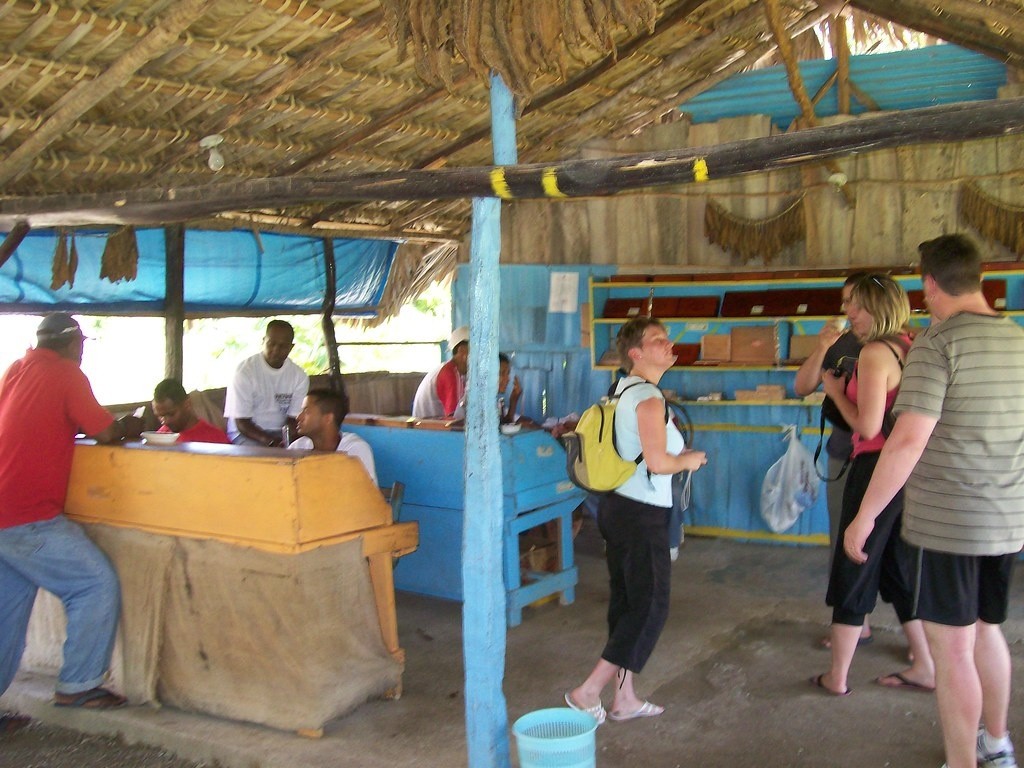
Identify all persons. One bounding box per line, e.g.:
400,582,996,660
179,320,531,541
844,233,1023,768
284,387,378,490
452,352,523,426
151,379,234,444
568,318,709,719
809,273,937,695
223,319,311,449
0,315,147,708
793,270,877,645
412,340,470,418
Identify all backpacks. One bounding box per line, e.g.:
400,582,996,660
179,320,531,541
562,381,670,491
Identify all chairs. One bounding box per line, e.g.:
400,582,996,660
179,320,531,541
378,481,409,570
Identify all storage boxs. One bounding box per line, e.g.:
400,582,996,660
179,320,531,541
699,324,834,400
515,538,579,607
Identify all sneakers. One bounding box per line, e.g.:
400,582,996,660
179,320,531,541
975,723,1016,768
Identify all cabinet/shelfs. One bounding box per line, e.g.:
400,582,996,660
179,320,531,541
586,262,1024,434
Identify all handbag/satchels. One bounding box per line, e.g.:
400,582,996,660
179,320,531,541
821,357,858,434
762,430,824,533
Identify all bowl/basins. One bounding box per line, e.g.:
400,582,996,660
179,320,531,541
140,431,181,444
501,423,521,435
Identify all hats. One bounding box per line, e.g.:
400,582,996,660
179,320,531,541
37,313,88,343
449,326,470,350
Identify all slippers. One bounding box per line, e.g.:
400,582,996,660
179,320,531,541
608,699,663,722
0,712,32,735
809,675,852,698
565,692,606,726
874,673,936,692
54,688,129,711
818,632,872,649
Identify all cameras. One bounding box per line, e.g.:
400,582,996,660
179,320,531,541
835,357,858,382
